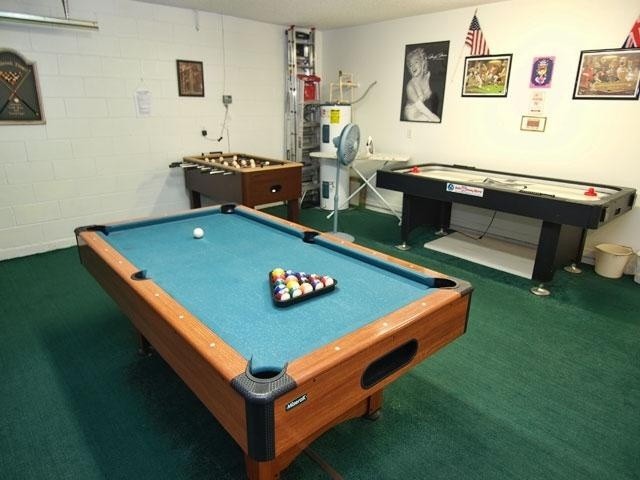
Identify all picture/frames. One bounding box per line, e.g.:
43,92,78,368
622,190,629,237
0,46,48,125
461,53,514,98
572,47,640,100
176,59,205,97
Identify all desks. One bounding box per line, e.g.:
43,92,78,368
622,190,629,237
374,160,637,297
73,203,476,480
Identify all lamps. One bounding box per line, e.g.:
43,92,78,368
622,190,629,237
0,0,97,29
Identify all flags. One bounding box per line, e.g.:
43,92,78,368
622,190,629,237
464,14,490,56
622,15,640,48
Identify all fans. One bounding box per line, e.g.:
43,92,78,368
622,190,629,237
323,123,360,243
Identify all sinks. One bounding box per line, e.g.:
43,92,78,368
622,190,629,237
349,156,408,179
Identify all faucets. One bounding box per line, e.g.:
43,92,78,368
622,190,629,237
366,135,374,156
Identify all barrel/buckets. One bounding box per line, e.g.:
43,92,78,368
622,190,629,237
593,243,633,279
633,250,640,284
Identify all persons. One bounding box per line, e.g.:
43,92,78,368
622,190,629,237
403,48,441,122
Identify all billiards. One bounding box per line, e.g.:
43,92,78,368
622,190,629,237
271,268,334,301
193,228,204,238
